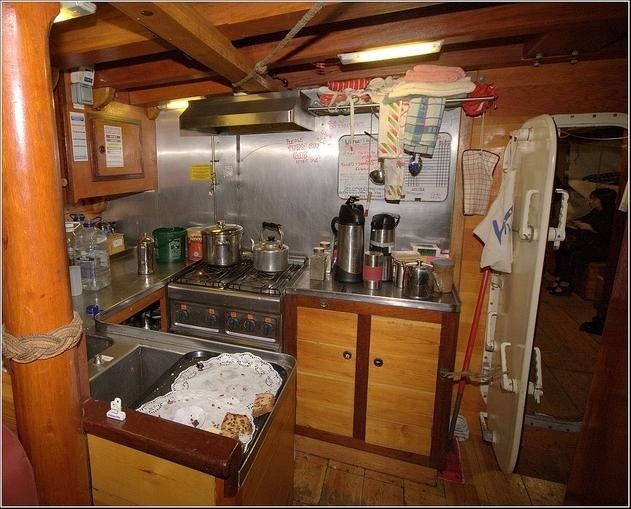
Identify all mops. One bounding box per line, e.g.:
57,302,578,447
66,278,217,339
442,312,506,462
437,267,491,485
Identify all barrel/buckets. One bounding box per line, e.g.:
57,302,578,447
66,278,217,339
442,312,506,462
152,226,187,263
68,212,113,293
186,227,206,263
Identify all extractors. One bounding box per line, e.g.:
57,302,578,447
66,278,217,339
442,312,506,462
178,89,317,137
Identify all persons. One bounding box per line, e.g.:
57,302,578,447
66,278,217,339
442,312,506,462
546,188,616,294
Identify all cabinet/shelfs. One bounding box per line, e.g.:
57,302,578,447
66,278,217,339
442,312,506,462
286,293,457,472
90,366,293,506
54,73,157,205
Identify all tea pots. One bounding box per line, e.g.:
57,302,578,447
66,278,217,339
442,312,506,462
331,195,366,284
250,221,289,273
368,213,402,282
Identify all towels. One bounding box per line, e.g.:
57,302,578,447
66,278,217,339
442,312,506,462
308,64,476,201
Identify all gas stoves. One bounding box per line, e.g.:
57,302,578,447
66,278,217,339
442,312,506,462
167,252,309,353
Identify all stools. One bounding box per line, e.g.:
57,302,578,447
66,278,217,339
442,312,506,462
578,262,612,303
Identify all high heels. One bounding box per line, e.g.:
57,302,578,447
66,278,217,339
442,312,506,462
546,280,572,296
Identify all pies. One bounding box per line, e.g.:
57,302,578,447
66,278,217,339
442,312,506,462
220,412,252,444
252,394,275,418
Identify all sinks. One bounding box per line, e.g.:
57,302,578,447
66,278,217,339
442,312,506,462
83,332,115,363
88,344,185,410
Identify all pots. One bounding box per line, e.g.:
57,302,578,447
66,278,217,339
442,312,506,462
200,219,245,268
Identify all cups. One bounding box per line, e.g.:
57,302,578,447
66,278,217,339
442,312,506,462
68,264,83,298
363,251,384,291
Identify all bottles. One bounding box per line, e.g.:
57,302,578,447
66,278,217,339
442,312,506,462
137,230,157,276
309,240,332,281
431,259,456,294
65,213,113,291
100,221,126,256
